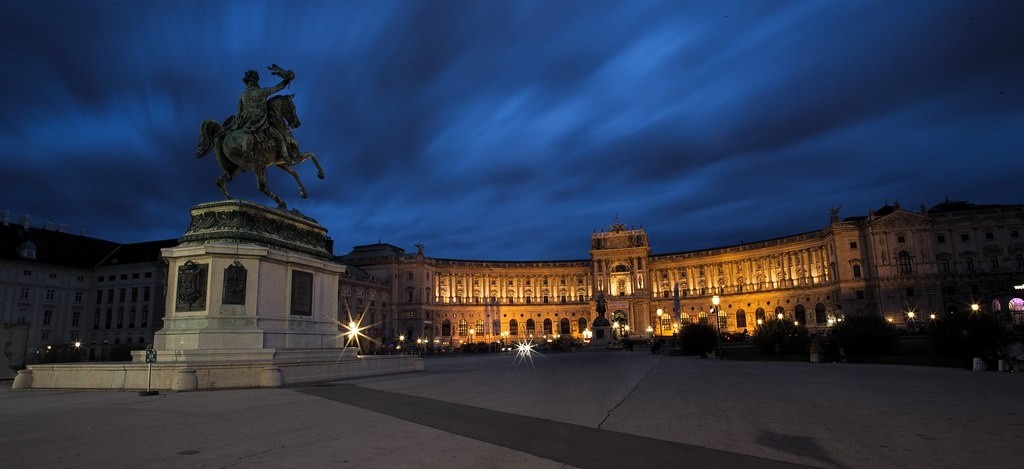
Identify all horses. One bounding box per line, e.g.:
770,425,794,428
196,93,324,211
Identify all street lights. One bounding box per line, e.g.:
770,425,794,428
656,308,665,351
500,330,511,345
711,294,722,339
907,312,917,333
469,328,475,344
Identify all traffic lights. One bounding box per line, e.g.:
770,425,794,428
620,323,625,337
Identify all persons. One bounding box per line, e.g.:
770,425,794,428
231,69,295,164
596,294,607,316
